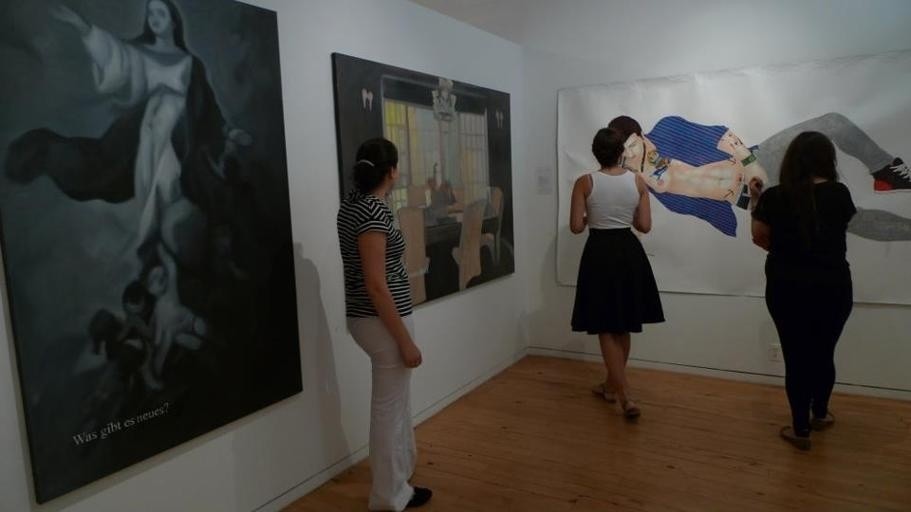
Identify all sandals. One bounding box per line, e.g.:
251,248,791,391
592,384,618,404
622,399,641,418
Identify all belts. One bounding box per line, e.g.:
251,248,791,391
737,185,750,210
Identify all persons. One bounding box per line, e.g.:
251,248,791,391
337,137,433,512
609,116,911,242
751,130,858,451
569,127,666,420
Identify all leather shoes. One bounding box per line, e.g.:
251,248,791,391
811,411,835,432
408,487,433,508
780,426,811,451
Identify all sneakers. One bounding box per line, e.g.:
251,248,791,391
872,158,911,192
